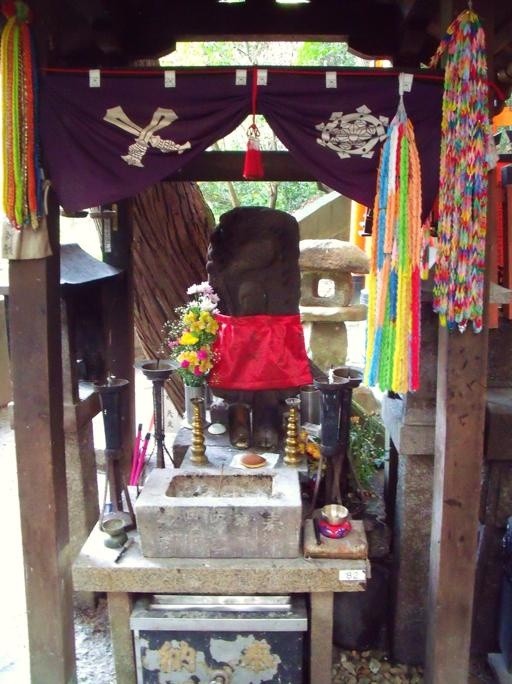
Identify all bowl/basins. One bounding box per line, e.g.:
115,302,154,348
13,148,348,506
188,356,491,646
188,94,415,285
321,502,350,526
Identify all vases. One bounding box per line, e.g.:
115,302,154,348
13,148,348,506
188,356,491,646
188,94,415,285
183,383,209,430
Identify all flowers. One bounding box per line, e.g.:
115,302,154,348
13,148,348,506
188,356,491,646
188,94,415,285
160,281,221,385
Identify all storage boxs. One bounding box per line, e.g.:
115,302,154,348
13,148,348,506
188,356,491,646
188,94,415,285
129,595,308,684
